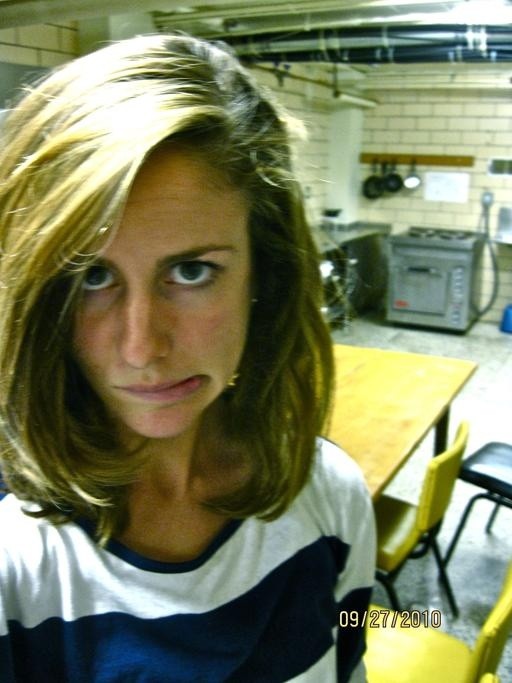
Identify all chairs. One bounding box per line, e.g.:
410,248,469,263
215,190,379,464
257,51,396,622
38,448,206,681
363,420,512,682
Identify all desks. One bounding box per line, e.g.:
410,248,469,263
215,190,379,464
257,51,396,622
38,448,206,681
315,345,480,503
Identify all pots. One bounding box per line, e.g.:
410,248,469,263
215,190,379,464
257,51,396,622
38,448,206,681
360,158,427,200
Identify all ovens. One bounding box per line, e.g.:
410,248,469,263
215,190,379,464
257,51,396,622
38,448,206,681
390,263,451,318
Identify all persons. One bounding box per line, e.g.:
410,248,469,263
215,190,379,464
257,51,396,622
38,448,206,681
0,32,383,681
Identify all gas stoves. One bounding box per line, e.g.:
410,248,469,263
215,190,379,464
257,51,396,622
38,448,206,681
404,226,473,240
